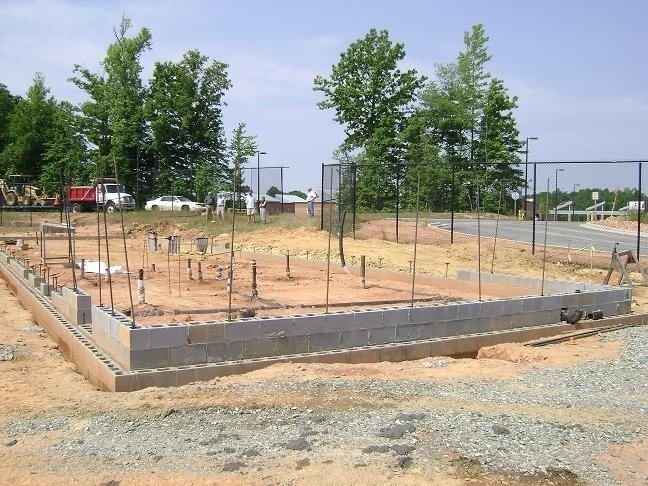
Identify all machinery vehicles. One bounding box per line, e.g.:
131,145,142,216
0,174,57,207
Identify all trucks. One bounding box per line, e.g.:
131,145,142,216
68,176,137,214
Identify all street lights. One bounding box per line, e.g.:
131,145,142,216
522,135,540,218
553,166,565,220
257,151,267,212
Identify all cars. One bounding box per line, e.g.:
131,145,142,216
144,195,206,213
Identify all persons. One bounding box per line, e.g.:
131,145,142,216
204,192,214,222
305,188,319,218
258,197,269,223
217,193,226,222
245,190,257,223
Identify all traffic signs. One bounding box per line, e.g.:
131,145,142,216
572,181,581,220
